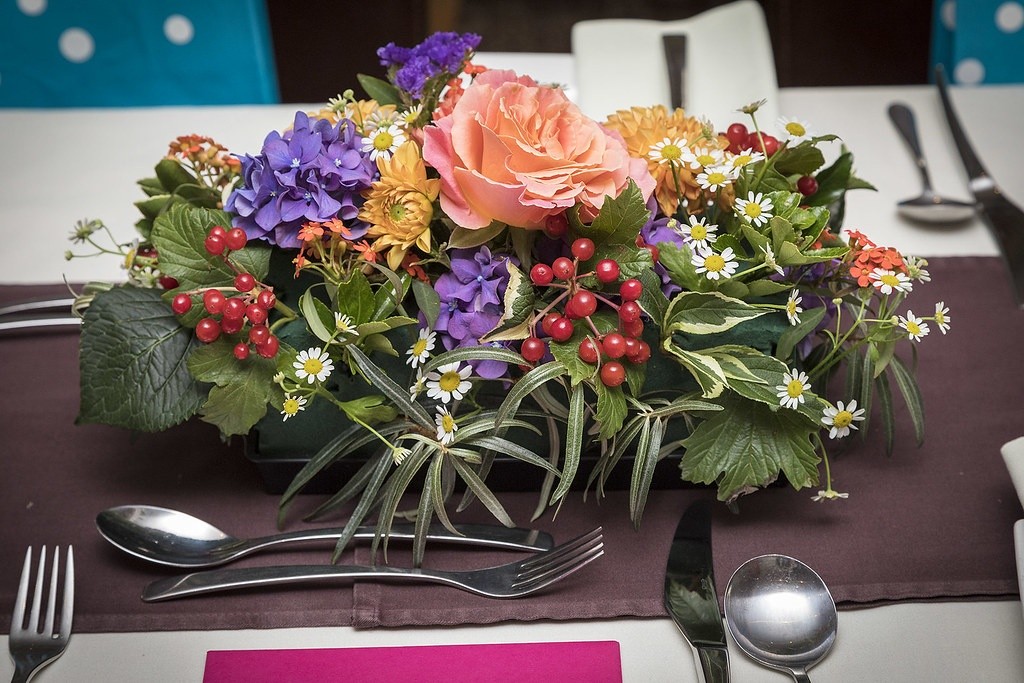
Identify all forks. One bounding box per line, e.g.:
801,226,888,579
9,544,75,683
142,525,605,604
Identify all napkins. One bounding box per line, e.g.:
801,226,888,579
469,1,781,138
0,255,1024,637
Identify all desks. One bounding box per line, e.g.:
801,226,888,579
0,85,1024,683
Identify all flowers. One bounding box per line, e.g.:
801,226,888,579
63,30,951,570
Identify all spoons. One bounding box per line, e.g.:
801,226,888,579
887,102,983,222
723,553,838,683
92,505,555,569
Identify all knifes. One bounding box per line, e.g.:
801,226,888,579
937,71,1024,306
663,501,732,683
